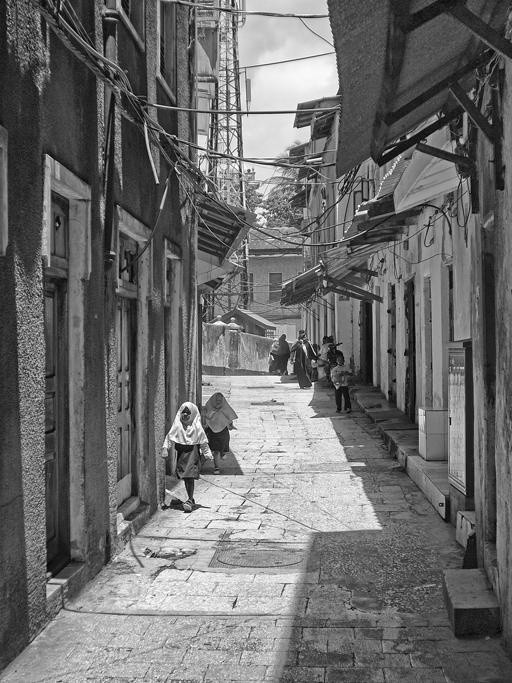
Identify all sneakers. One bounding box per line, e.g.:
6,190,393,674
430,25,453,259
213,454,227,474
335,406,351,413
183,498,196,511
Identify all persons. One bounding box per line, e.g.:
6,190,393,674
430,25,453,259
160,400,214,512
196,392,239,476
330,350,354,414
269,333,290,377
309,335,343,385
288,328,318,389
